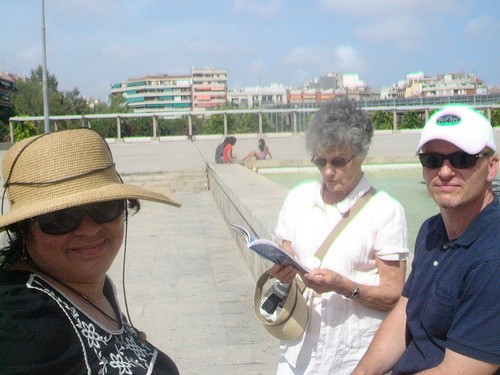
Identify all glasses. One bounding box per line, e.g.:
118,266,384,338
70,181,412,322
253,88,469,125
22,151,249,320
38,198,124,235
417,150,493,168
310,150,356,168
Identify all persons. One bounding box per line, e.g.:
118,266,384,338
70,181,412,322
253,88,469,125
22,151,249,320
0,130,185,375
223,137,239,165
350,103,500,375
243,139,273,161
269,99,410,375
215,137,230,163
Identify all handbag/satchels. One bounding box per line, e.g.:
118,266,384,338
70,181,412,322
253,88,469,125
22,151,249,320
254,267,311,342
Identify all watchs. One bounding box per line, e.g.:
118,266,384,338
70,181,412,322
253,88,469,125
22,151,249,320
349,282,359,299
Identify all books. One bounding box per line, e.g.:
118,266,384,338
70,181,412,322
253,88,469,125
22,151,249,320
229,222,311,275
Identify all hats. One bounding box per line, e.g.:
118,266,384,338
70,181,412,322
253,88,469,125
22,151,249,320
0,128,182,231
414,105,497,155
